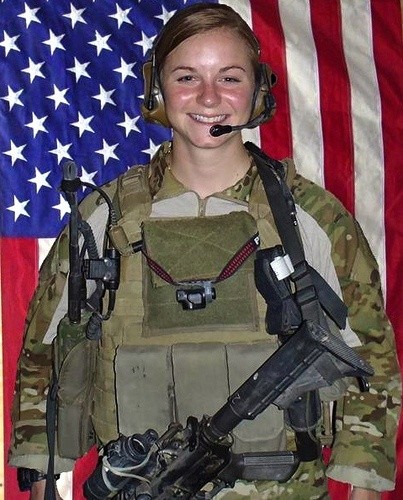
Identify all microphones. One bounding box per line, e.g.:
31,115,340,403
209,108,271,137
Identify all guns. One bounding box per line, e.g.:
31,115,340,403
83,318,375,500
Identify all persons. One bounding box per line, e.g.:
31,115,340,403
7,4,403,500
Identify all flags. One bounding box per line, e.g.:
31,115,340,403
0,0,403,500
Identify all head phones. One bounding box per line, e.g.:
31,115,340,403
138,3,278,130
